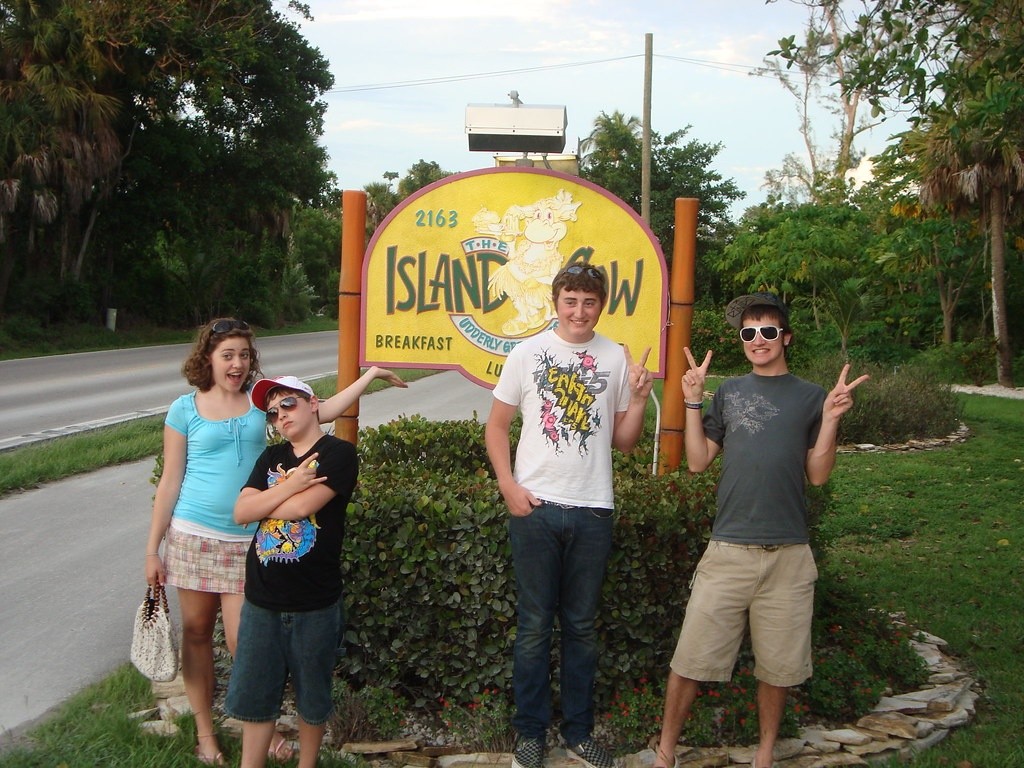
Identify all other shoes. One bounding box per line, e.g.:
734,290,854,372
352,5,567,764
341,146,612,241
566,737,618,768
512,736,543,768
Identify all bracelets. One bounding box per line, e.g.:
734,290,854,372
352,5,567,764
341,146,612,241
683,398,703,409
146,553,158,556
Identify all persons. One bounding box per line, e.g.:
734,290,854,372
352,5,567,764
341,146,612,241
223,375,359,768
653,291,869,768
485,261,653,768
145,318,409,766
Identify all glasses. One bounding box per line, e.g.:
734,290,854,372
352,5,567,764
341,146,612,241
562,265,604,282
739,325,784,341
265,396,301,423
209,320,250,337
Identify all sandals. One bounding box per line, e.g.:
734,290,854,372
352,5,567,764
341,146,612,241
267,736,294,765
197,732,225,768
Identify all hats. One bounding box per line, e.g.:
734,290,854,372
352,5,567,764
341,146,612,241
725,291,788,329
251,376,314,412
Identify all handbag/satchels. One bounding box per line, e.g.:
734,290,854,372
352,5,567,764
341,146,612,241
131,581,179,682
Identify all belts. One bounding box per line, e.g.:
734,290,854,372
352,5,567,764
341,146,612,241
721,541,794,553
543,501,580,510
